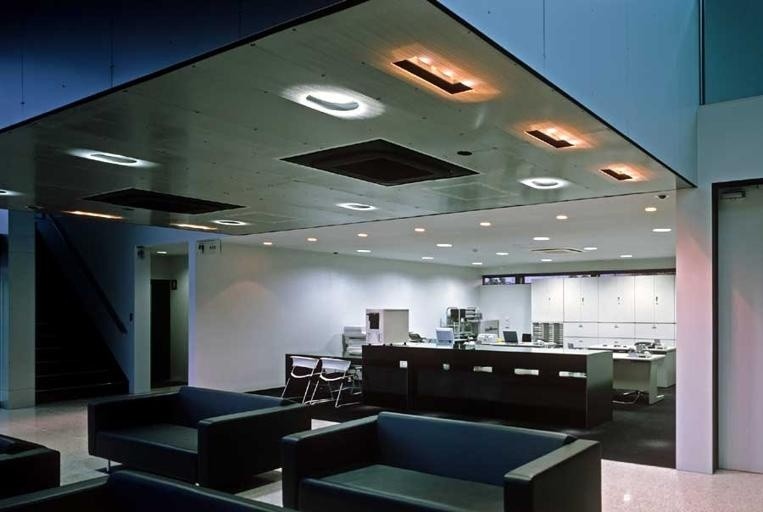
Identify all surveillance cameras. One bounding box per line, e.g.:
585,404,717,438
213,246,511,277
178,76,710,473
655,193,669,200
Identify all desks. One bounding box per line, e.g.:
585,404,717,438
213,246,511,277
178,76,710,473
590,346,677,390
279,351,362,403
611,354,665,408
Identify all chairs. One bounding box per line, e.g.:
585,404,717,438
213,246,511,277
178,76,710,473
279,354,321,405
309,356,360,407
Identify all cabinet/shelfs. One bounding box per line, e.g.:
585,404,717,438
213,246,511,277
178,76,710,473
445,306,479,343
359,337,614,429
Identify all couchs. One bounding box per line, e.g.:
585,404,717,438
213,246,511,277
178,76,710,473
2,467,291,512
279,405,601,512
0,428,62,497
81,383,311,491
